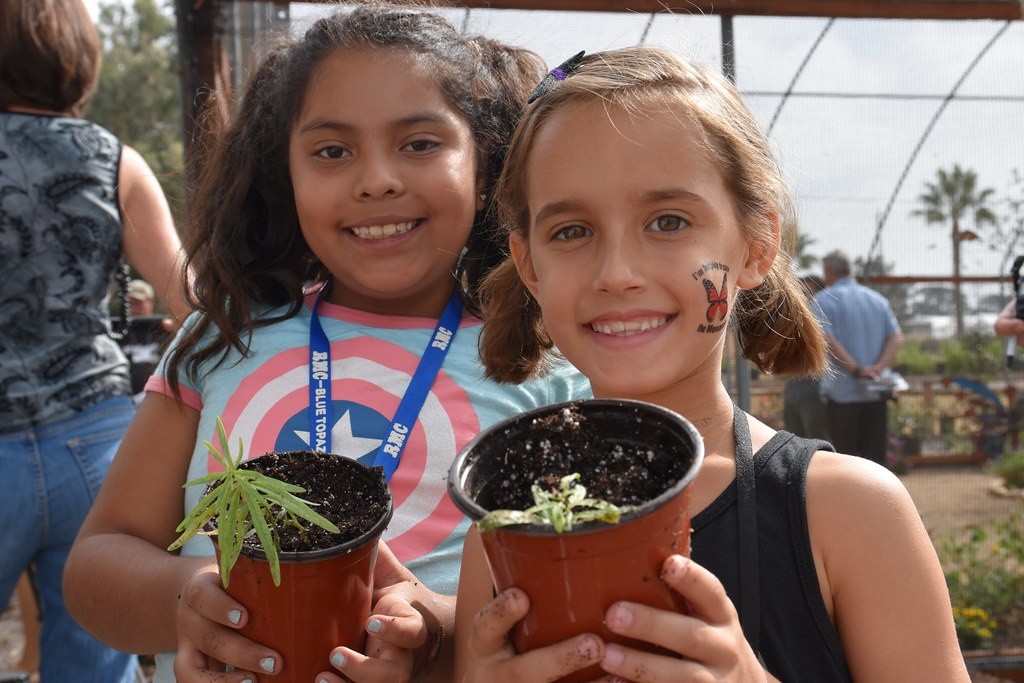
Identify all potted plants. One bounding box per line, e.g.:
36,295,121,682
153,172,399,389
167,416,394,683
447,398,708,655
941,378,1024,459
896,408,935,455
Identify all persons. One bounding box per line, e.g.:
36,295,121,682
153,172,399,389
0,2,203,683
445,43,969,683
781,251,905,467
994,254,1024,351
63,9,595,681
